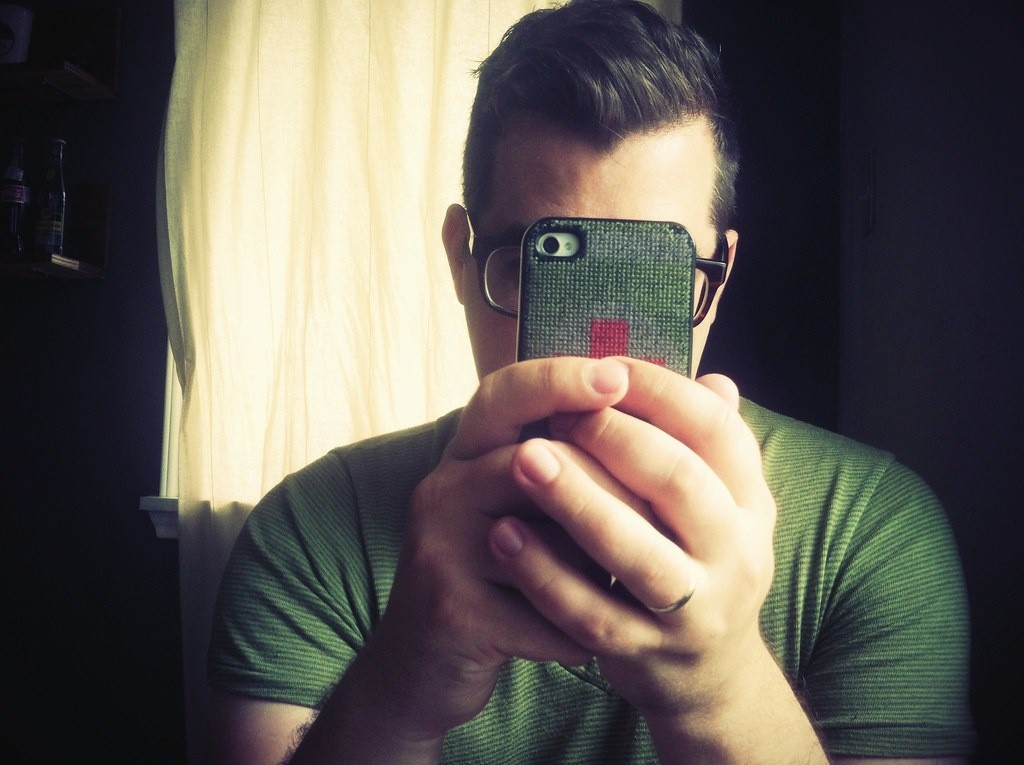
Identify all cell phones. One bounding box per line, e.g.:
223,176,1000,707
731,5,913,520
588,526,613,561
515,216,695,592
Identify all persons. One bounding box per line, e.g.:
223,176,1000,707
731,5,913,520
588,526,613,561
207,0,981,765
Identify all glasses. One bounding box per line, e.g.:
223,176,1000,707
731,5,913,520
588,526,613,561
464,208,730,329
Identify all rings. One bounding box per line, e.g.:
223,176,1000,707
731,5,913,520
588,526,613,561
648,554,699,612
